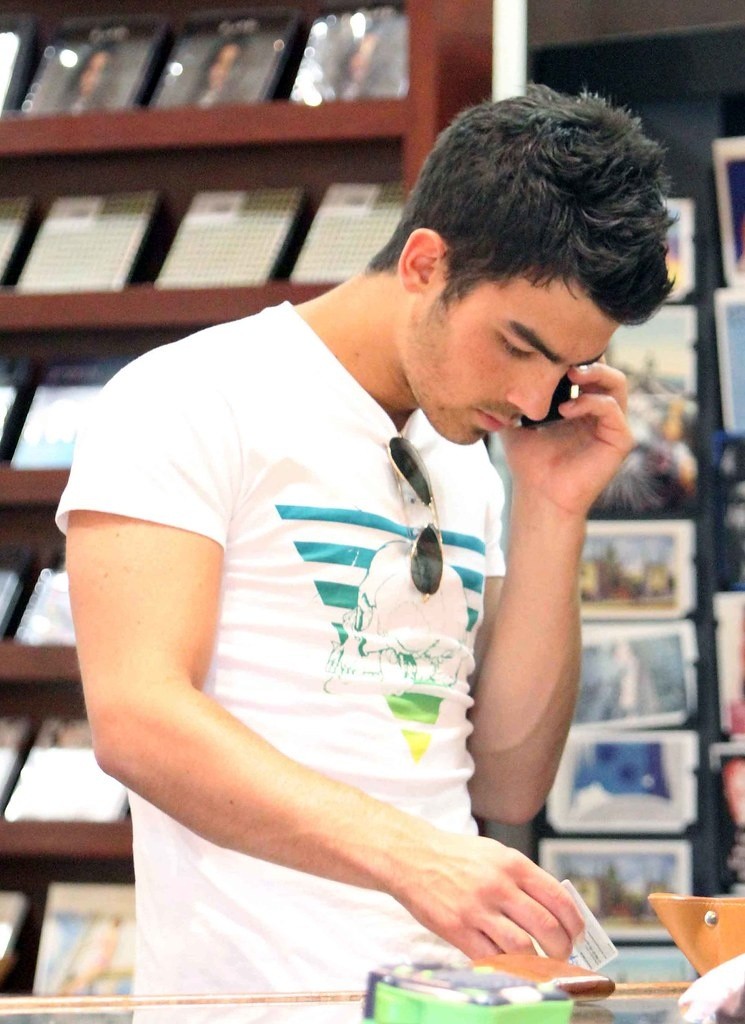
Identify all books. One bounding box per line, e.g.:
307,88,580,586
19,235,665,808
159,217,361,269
0,534,79,648
22,11,410,116
0,713,130,823
0,353,123,468
0,880,137,995
0,182,405,294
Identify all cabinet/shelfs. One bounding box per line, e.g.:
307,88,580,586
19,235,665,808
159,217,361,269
0,1,496,995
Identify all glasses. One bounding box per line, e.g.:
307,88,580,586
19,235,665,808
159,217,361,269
387,434,445,605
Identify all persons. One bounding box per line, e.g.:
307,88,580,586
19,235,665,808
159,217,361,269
67,45,111,108
331,21,392,97
193,36,251,106
56,93,672,997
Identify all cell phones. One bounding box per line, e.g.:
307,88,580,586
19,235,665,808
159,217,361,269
520,371,579,428
